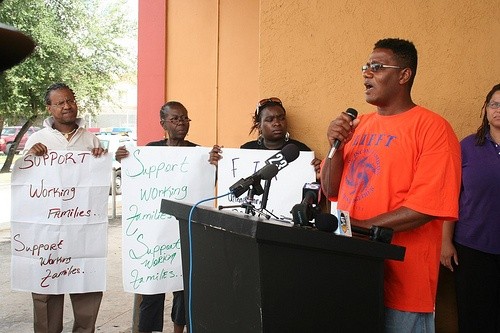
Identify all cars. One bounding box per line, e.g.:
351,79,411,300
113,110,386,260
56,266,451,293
86,126,134,137
0,126,35,154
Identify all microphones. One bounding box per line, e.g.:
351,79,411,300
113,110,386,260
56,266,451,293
315,210,394,244
229,144,300,191
233,164,278,198
292,182,322,224
328,108,358,159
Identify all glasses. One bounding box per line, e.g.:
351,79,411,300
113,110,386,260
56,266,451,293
161,118,191,125
362,63,408,74
257,97,283,115
485,101,500,109
49,99,76,107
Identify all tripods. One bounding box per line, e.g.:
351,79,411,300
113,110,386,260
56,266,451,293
218,189,270,220
253,179,280,220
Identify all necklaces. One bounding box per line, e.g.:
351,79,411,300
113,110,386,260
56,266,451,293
53,125,78,139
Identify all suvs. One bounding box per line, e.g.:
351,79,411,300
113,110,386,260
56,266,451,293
96,135,136,194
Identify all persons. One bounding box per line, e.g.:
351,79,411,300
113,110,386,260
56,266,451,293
320,38,462,333
439,83,500,333
115,103,217,333
22,83,107,333
209,98,324,208
